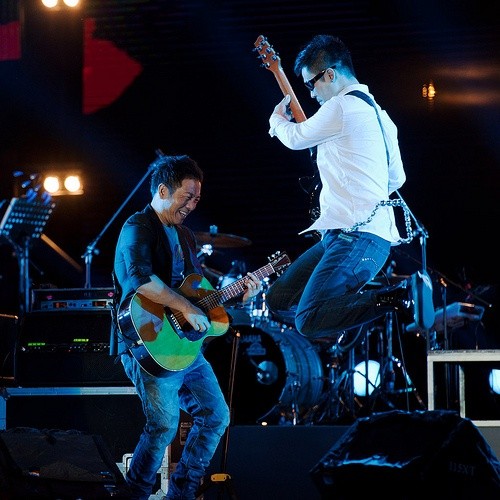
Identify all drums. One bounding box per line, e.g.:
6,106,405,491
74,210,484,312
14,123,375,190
202,322,324,425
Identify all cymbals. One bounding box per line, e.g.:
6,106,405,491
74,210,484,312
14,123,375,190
194,231,251,247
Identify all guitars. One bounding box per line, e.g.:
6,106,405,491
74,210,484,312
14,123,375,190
117,248,292,378
252,34,323,240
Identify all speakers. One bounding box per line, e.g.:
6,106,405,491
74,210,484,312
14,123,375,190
14,290,137,386
0,430,134,500
308,411,500,500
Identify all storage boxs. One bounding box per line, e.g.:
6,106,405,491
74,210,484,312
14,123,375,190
427,350,500,426
0,385,169,500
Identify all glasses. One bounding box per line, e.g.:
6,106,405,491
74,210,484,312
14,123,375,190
304,65,337,90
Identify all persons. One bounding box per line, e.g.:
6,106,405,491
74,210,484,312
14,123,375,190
263,34,437,337
110,152,264,500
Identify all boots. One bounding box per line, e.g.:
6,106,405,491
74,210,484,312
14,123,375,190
375,270,436,332
337,326,367,353
